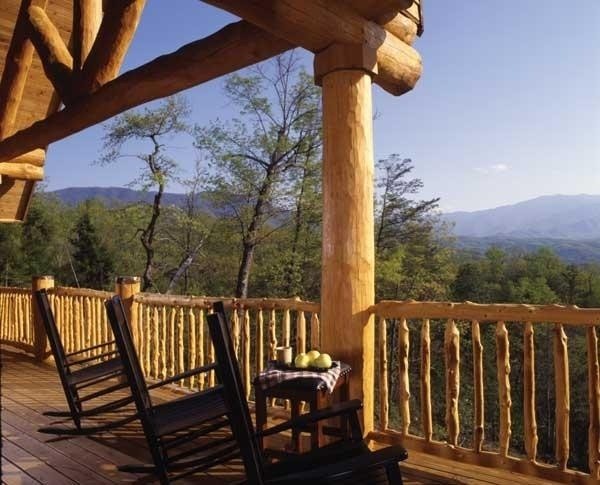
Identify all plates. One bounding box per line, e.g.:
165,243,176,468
268,359,339,373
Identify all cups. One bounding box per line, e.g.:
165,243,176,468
274,346,293,365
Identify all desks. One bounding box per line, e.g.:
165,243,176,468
253,359,350,457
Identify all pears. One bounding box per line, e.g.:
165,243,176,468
294,352,307,369
315,353,332,369
307,349,320,367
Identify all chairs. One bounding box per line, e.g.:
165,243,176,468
36,286,142,434
204,300,407,485
101,292,244,485
